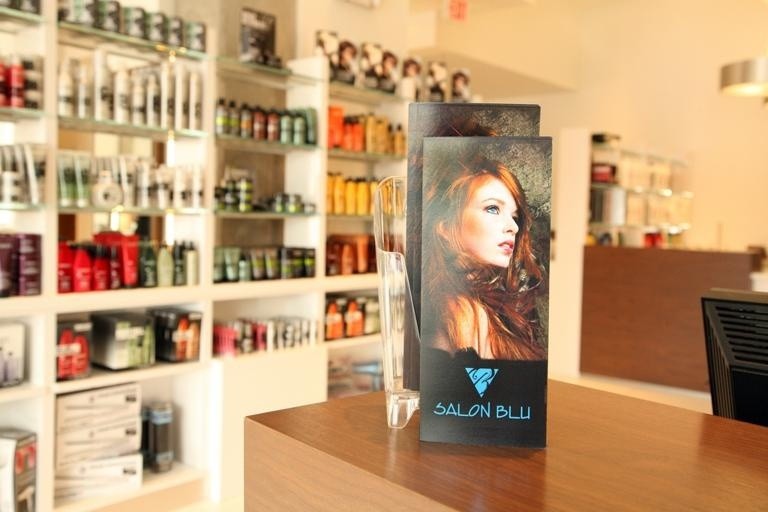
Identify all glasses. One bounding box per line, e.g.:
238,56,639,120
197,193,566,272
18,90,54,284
716,59,768,98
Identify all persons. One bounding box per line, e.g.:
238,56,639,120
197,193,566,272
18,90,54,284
423,152,548,360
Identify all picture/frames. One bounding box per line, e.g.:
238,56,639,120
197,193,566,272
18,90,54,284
244,375,768,512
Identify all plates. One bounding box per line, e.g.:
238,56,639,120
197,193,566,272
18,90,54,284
215,32,328,507
0,1,53,511
51,0,211,512
324,56,417,398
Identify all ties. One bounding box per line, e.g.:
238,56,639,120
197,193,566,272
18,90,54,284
700,287,767,427
